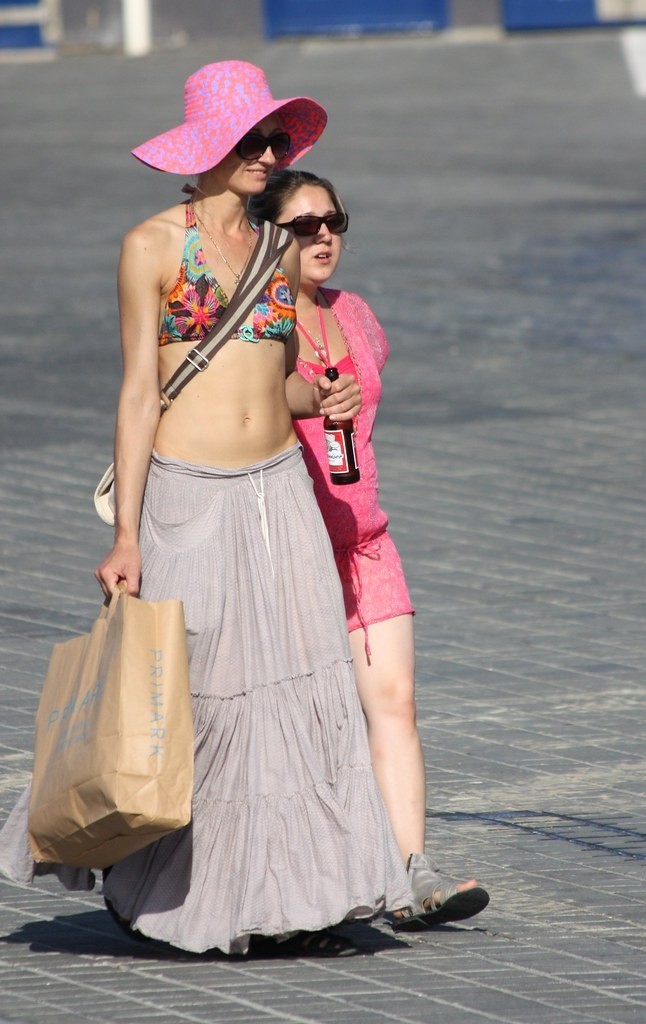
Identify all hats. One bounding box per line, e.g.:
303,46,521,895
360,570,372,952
129,61,328,177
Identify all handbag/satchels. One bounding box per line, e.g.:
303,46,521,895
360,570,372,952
93,459,118,527
27,578,194,868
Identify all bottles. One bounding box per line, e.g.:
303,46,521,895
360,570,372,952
322,368,360,485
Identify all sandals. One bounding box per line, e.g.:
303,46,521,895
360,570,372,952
390,858,489,929
251,930,357,957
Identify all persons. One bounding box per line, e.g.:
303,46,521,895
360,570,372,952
96,58,417,959
253,169,489,933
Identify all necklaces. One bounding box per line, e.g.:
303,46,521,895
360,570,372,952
294,295,327,360
196,210,254,288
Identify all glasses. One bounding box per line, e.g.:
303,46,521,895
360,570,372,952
235,133,293,161
261,212,349,237
